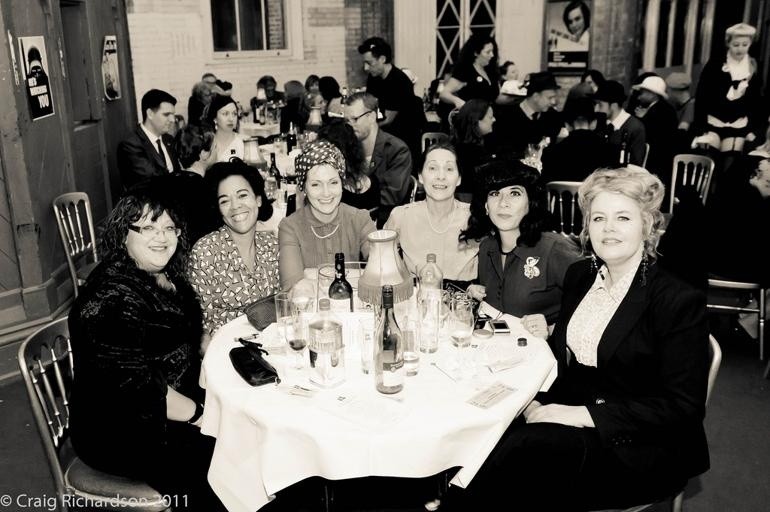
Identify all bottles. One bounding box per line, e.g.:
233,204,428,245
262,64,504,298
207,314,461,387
417,253,442,354
423,86,428,111
236,99,280,125
228,149,237,160
287,122,297,156
375,285,404,393
329,250,354,312
307,298,344,388
616,127,632,167
268,152,281,189
341,87,348,103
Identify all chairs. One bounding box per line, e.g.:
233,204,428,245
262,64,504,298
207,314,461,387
171,109,770,362
18,315,180,512
618,330,723,512
48,188,109,300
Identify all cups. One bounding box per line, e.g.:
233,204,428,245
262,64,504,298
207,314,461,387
274,180,288,208
419,297,440,355
395,320,421,376
273,291,307,371
273,138,283,153
316,265,349,311
350,319,379,384
447,291,475,351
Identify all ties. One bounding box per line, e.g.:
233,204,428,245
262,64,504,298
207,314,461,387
157,139,167,168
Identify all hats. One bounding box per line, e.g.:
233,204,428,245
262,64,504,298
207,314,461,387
524,71,560,92
584,79,627,102
632,76,669,100
666,71,691,90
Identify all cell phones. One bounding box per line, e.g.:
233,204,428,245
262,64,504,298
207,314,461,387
489,320,511,333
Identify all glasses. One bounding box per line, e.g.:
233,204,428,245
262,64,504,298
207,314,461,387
128,223,182,237
345,110,372,122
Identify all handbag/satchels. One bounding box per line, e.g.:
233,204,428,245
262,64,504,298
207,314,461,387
229,338,280,386
244,292,300,331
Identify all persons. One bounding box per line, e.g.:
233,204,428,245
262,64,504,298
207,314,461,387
563,2,589,46
70,23,770,510
